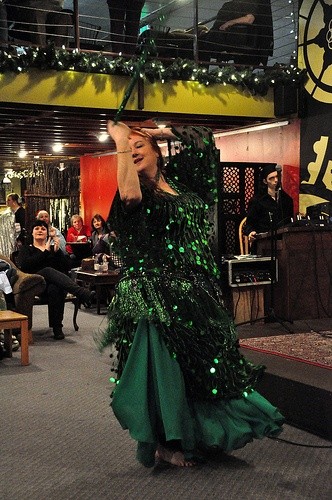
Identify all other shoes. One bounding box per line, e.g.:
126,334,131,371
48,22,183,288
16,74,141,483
53,327,64,340
85,290,96,302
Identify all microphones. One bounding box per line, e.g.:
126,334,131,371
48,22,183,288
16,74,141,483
275,165,282,190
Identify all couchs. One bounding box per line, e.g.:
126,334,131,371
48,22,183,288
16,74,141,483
0,254,46,344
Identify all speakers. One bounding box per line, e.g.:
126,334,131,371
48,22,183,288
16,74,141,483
275,84,297,116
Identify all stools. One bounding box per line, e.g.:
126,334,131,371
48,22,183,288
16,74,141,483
0,310,30,366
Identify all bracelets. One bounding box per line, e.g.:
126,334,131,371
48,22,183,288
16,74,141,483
117,148,132,155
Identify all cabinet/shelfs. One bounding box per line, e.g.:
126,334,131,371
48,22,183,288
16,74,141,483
225,287,264,326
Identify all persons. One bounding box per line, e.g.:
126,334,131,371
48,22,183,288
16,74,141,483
0,192,121,353
103,117,287,468
243,166,294,257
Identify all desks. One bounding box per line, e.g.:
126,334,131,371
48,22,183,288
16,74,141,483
65,242,93,261
73,270,120,332
254,226,332,321
